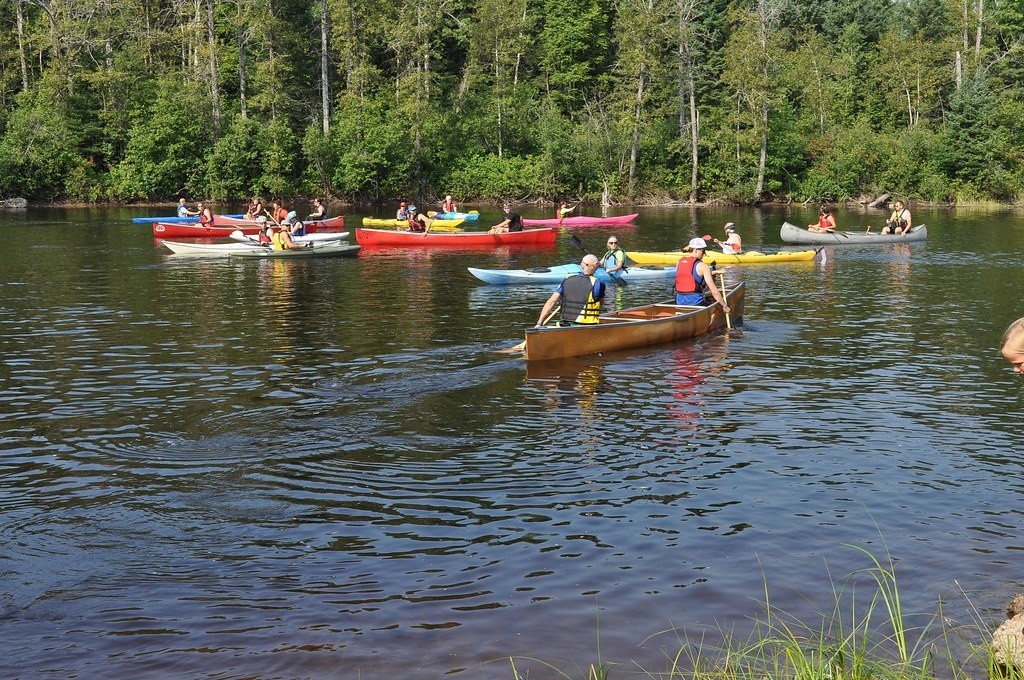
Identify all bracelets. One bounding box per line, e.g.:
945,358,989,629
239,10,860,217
536,321,543,326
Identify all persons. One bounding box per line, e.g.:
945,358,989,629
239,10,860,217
442,195,457,214
273,220,309,251
487,203,522,235
176,198,199,217
194,201,214,227
674,238,731,315
533,253,606,328
556,201,577,219
598,236,626,278
267,200,288,224
881,200,912,238
256,215,274,247
407,205,432,238
713,222,742,254
243,196,263,220
396,202,407,221
807,205,837,234
286,210,306,236
308,197,329,221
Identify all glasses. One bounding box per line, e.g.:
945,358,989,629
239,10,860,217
285,224,290,227
504,207,509,210
609,242,616,245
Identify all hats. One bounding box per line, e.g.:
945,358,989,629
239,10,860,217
281,220,291,225
409,207,416,211
690,238,707,248
582,254,598,268
401,202,406,206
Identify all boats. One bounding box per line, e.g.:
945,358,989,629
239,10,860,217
150,220,281,238
228,246,362,258
780,221,927,245
466,263,677,284
625,245,825,265
427,210,481,222
525,279,747,362
132,215,244,225
211,214,346,228
230,230,351,243
360,217,465,228
354,224,557,245
522,213,640,226
161,240,342,254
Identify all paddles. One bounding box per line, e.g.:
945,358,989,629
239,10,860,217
810,226,849,239
264,209,282,227
232,230,260,246
568,196,588,216
720,273,732,329
513,306,561,350
702,234,741,251
569,234,627,286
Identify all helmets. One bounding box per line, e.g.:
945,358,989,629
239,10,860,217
724,222,734,229
258,216,268,223
287,211,297,219
